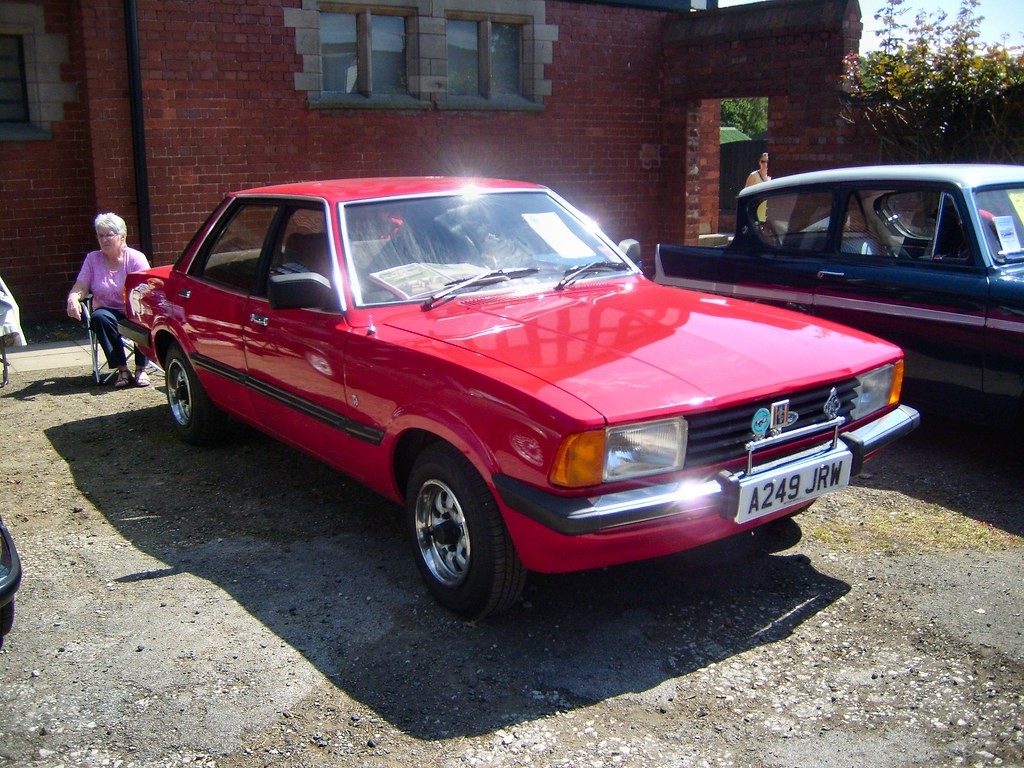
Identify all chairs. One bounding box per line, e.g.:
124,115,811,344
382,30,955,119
81,293,167,384
285,230,332,280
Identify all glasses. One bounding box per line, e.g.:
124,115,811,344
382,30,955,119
760,159,768,164
97,231,117,240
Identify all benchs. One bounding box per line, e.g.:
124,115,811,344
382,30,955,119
781,236,889,262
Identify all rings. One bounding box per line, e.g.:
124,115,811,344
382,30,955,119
70,313,73,315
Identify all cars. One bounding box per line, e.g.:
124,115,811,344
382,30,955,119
116,176,921,625
655,164,1023,412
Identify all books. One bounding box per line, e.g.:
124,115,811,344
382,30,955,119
367,261,503,303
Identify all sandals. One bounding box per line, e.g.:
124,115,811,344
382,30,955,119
134,369,150,386
115,369,132,388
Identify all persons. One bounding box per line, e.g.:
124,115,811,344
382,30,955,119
744,152,771,224
139,277,201,335
65,211,154,388
